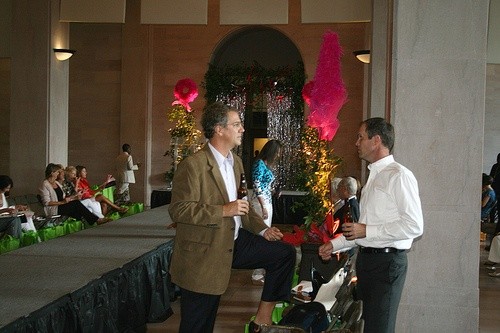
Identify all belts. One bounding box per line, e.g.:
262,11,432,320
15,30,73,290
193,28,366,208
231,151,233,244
359,246,405,253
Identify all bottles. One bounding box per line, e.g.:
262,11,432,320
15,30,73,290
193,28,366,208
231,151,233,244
344,198,355,240
237,174,248,201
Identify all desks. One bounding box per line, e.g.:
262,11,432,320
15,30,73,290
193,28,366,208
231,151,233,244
151,189,171,209
278,189,312,223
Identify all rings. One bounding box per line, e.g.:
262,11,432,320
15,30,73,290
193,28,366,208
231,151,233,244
274,239,277,241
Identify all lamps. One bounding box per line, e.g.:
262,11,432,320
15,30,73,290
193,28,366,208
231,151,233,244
352,49,370,63
54,47,76,59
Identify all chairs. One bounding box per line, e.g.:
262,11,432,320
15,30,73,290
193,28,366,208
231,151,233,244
244,244,365,332
0,184,144,254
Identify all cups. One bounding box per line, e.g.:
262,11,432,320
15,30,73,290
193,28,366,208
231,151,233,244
46,206,52,218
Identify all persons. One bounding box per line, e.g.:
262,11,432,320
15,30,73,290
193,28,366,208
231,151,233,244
114,143,142,202
167,102,298,333
249,140,283,287
481,153,500,239
0,174,42,245
291,176,360,294
319,117,424,333
41,163,132,227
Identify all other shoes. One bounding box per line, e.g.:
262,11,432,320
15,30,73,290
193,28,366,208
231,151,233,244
98,218,113,225
248,322,277,333
119,207,128,212
489,272,500,276
484,261,498,267
